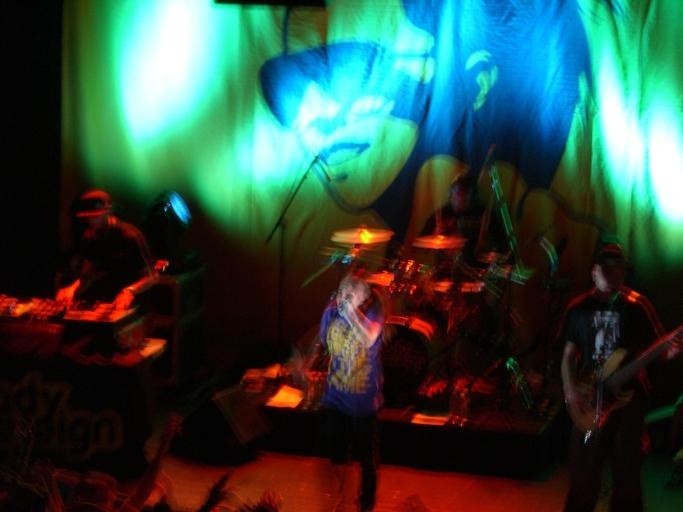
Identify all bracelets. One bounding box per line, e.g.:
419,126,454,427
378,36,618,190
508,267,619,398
124,285,136,296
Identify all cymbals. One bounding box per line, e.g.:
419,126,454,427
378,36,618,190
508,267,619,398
330,229,395,243
412,234,470,250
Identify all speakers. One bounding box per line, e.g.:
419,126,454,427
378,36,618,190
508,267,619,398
167,385,275,467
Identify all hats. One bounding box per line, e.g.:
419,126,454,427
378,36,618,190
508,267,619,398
591,241,626,261
73,190,112,218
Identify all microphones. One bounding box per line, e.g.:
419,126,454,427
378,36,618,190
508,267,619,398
611,290,623,303
337,293,355,310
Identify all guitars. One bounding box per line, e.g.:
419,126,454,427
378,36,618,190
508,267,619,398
567,324,682,438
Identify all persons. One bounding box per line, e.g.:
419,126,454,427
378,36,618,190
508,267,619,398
560,242,681,511
315,274,381,511
52,189,163,310
396,162,517,276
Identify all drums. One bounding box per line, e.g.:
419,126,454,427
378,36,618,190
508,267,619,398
379,318,433,371
